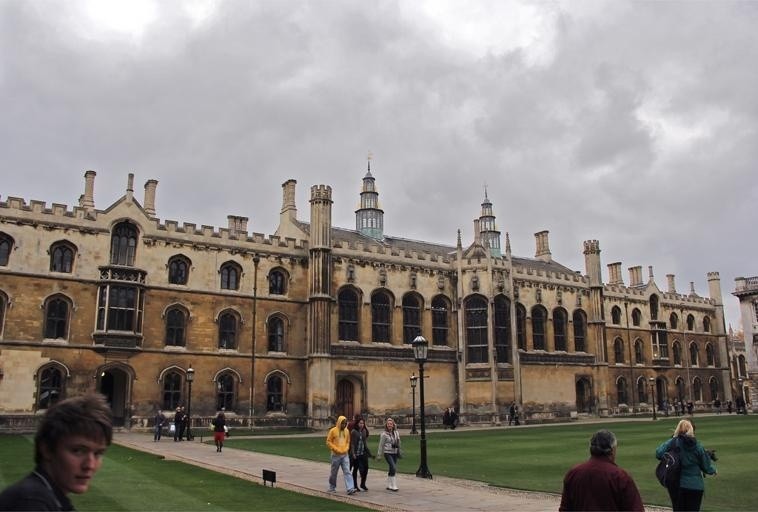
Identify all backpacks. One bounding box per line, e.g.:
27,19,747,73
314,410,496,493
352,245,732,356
656,436,682,490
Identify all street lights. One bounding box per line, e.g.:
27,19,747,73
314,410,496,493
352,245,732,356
410,372,418,435
649,376,658,420
738,377,743,414
186,362,196,440
414,332,434,480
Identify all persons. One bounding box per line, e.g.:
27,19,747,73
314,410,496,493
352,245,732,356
153,404,231,452
349,418,375,492
0,392,113,512
326,415,358,496
509,403,516,425
443,407,458,429
559,430,645,512
656,419,717,512
514,406,520,425
662,397,746,418
376,418,402,491
347,414,369,472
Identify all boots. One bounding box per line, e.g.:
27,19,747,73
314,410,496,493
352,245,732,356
385,475,399,491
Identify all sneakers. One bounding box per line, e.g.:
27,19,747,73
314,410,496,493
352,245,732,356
327,484,370,495
